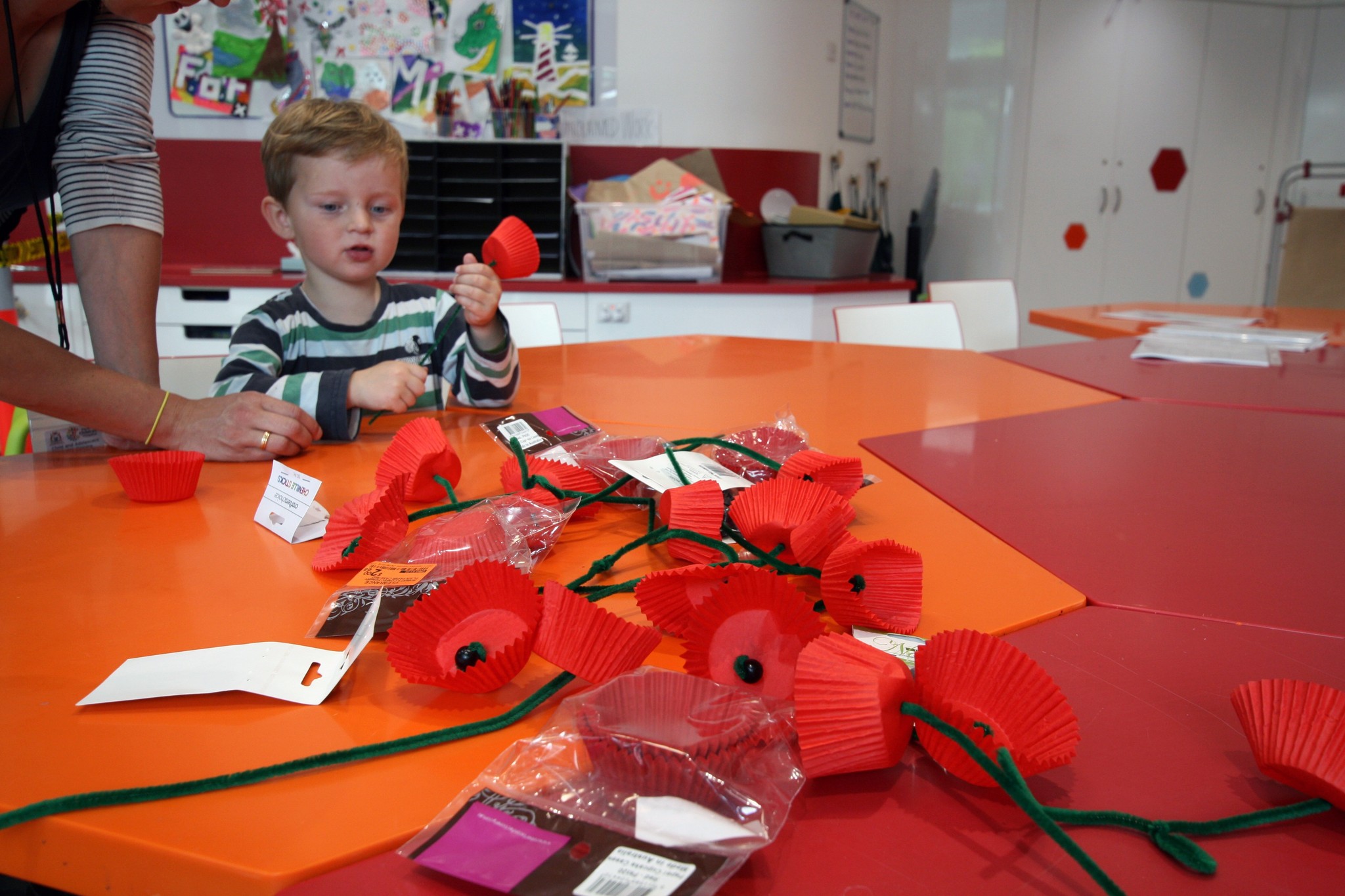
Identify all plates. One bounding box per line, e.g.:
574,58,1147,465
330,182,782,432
761,189,797,225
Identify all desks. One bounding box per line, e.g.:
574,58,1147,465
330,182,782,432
0,303,1345,896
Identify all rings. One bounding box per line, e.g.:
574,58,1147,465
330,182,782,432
260,431,270,450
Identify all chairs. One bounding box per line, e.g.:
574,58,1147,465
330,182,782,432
833,280,1018,352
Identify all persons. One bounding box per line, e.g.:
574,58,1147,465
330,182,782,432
0,0,323,462
209,98,521,440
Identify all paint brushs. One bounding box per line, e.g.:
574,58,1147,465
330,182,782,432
553,93,572,114
482,76,529,137
533,93,556,114
433,88,458,137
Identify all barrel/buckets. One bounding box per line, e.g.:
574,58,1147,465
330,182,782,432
762,221,880,279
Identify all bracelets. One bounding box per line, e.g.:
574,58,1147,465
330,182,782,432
145,391,170,445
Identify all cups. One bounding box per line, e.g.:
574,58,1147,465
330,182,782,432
437,113,453,138
491,108,535,139
